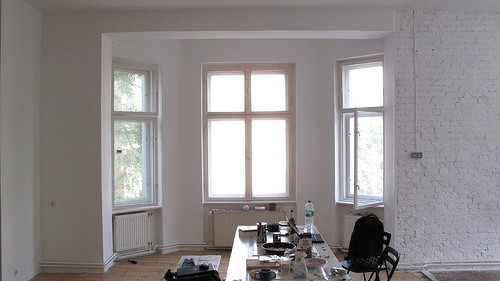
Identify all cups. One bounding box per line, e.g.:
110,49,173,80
254,268,272,281
256,222,267,243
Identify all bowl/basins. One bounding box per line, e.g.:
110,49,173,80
304,258,327,270
260,242,296,257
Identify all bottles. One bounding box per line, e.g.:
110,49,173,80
298,235,312,259
304,200,315,233
288,210,296,234
278,220,291,235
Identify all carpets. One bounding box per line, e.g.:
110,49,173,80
173,255,221,279
421,268,500,281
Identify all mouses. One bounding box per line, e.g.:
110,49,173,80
199,264,209,270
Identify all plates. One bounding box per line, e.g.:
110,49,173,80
249,270,276,280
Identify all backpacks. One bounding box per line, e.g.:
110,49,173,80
347,214,384,269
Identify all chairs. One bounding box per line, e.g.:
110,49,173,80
340,230,401,281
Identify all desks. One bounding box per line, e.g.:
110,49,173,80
225,224,352,281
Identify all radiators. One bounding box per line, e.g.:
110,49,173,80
113,212,148,251
208,208,286,247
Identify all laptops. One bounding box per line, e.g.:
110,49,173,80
286,216,325,243
177,262,215,279
